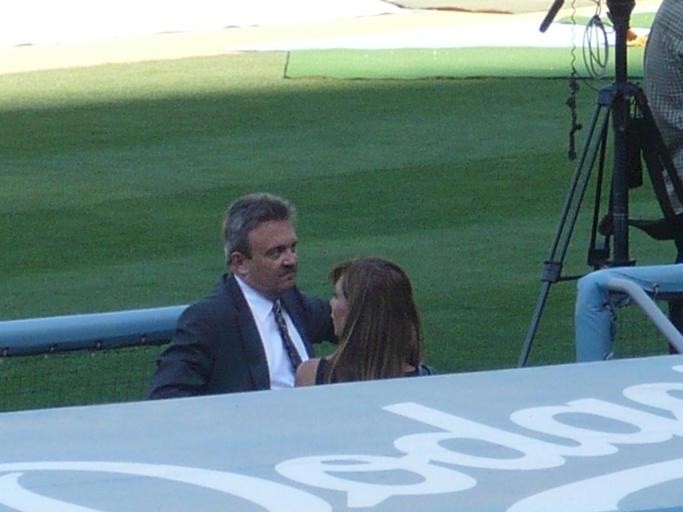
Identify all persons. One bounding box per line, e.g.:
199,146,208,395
147,192,339,399
295,258,435,388
643,0,683,211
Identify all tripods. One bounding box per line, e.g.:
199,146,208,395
517,0,683,368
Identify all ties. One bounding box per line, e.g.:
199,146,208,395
272,298,302,369
